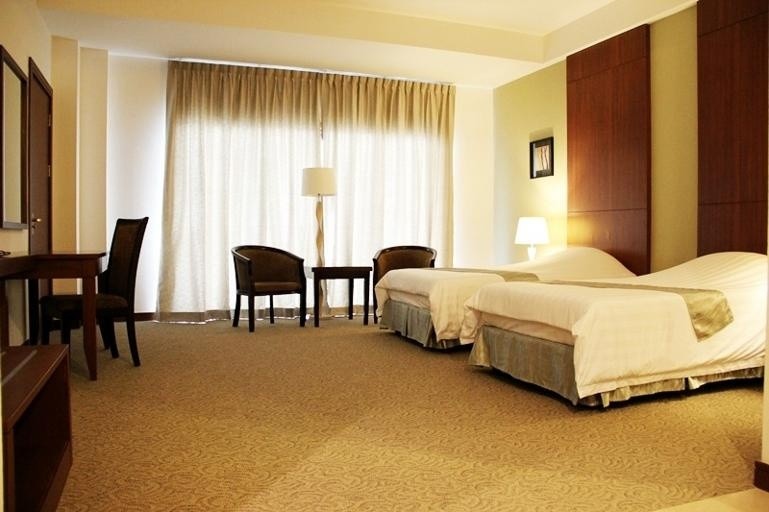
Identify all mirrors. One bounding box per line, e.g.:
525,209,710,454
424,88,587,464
0,43,29,230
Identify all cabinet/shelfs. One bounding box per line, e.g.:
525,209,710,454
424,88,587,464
0,341,73,511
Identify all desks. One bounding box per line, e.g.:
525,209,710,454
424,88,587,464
1,251,109,380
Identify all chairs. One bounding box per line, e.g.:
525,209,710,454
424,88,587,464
39,216,148,367
231,245,306,332
372,245,437,323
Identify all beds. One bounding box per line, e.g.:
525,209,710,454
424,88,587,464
457,251,768,410
373,246,637,354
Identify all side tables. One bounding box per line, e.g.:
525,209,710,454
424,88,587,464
311,266,372,327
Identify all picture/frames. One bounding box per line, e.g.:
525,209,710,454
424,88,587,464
529,136,554,179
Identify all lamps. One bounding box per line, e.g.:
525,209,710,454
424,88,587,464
515,216,549,261
300,167,338,321
